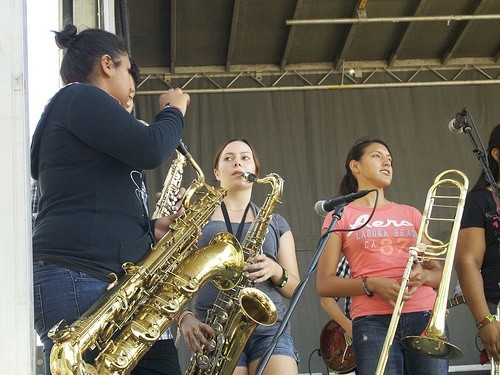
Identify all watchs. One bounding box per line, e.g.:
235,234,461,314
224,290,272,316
162,102,184,113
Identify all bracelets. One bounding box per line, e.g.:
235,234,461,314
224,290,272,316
174,306,192,326
476,314,496,329
361,276,375,297
178,311,195,327
274,267,289,288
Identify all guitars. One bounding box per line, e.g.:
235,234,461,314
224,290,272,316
318,292,466,374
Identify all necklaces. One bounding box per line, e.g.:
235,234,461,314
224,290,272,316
227,207,244,212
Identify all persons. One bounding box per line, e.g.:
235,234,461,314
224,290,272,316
173,138,301,375
317,124,500,375
32,24,190,375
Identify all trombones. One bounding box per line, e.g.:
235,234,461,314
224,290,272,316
374,169,464,375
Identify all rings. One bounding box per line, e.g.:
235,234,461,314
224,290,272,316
195,329,200,335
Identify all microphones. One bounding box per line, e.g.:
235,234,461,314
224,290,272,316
314,189,369,217
448,107,467,134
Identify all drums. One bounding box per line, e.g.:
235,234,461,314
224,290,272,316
185,170,285,375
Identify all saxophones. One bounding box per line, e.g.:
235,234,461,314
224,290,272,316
45,119,244,375
